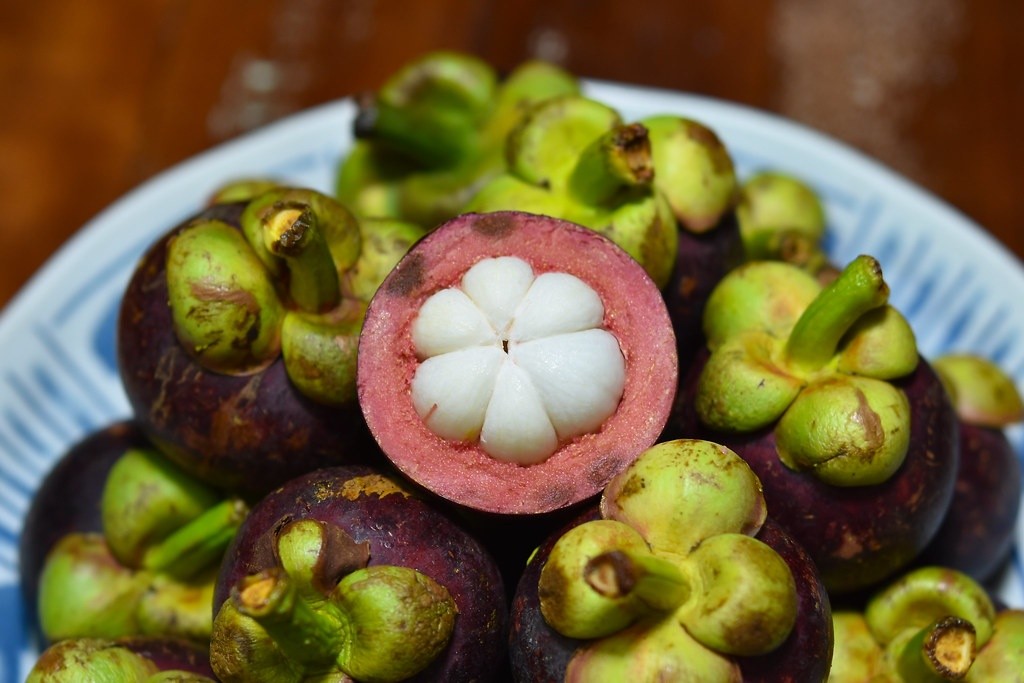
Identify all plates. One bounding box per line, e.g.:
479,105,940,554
0,81,1024,683
